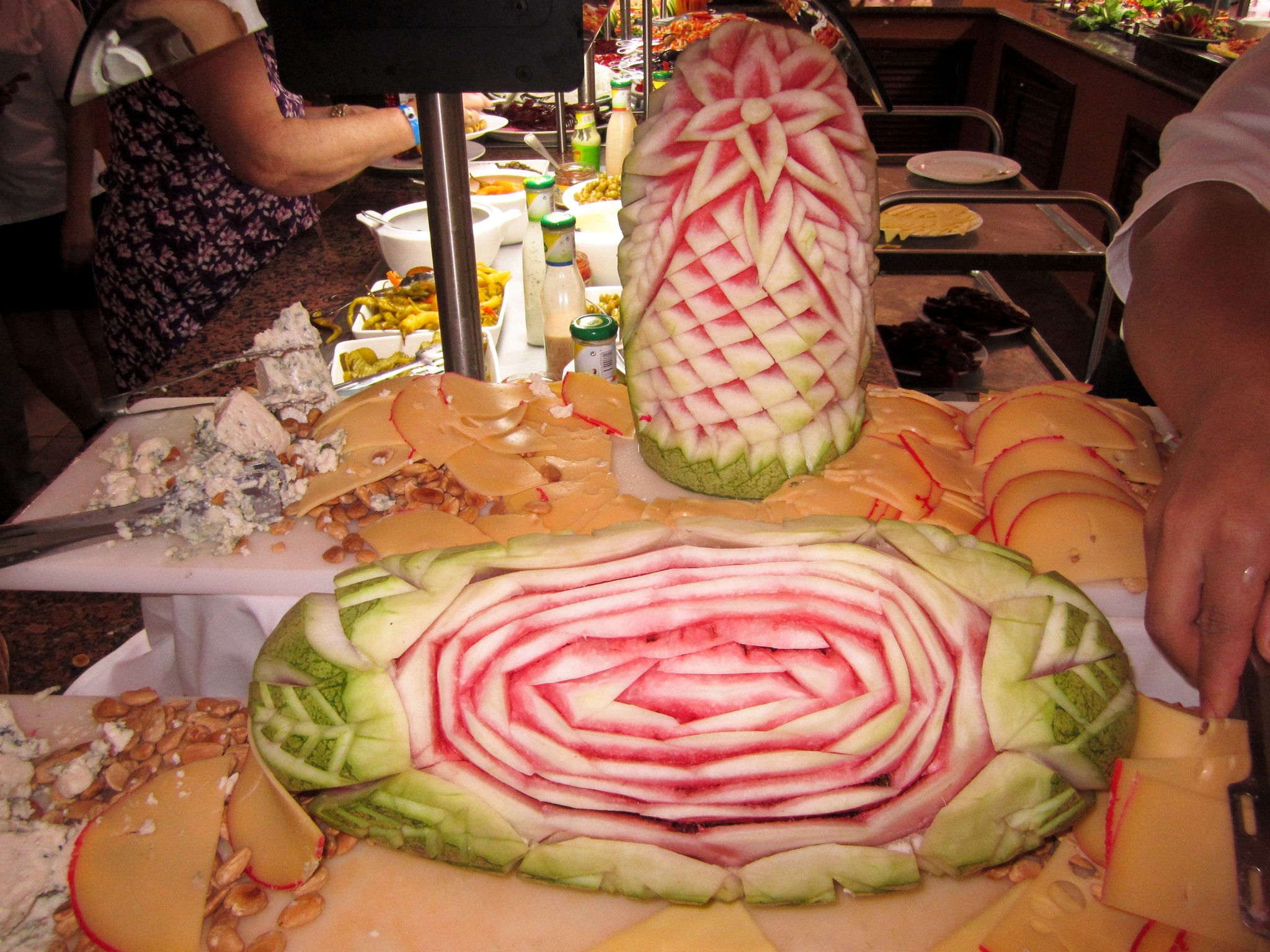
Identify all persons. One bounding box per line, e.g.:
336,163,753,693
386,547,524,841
1103,34,1270,734
0,1,494,449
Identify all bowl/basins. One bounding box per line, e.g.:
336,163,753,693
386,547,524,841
356,201,522,279
331,330,502,396
351,277,509,348
564,200,621,287
469,169,542,246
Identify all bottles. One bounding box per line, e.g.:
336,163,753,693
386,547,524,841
502,77,637,392
652,70,670,91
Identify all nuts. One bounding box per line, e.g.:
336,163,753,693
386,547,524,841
31,689,362,951
165,407,565,568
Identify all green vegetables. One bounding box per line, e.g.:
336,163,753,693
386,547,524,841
1069,0,1211,38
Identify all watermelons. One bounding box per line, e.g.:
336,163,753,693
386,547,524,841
614,20,882,497
248,521,1139,906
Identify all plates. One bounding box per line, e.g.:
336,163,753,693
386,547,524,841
465,114,508,141
1208,49,1236,61
906,150,1021,185
915,295,1030,336
562,176,614,210
369,140,486,173
615,37,644,97
468,159,550,175
1138,17,1246,46
585,286,623,350
880,209,983,238
485,124,608,144
893,336,987,378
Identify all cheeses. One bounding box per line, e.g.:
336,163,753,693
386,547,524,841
69,754,232,951
225,714,324,891
586,692,1264,952
297,371,1172,592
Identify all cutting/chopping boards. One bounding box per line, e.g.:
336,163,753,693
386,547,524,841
1,396,1148,620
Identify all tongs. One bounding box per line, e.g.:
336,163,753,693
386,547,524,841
96,343,327,423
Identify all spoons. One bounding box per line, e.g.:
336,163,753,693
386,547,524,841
309,266,436,320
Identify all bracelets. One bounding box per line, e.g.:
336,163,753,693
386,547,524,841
331,104,346,118
398,105,421,147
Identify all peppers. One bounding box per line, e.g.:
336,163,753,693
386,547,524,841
313,264,511,380
469,173,524,197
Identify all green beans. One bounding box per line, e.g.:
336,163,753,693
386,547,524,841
584,293,620,327
574,172,622,206
